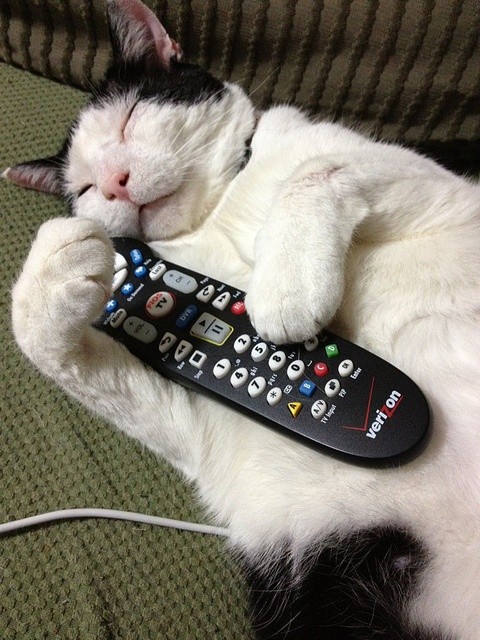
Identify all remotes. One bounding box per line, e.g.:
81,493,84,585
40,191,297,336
82,236,430,467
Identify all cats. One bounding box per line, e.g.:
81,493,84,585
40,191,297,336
0,2,480,640
